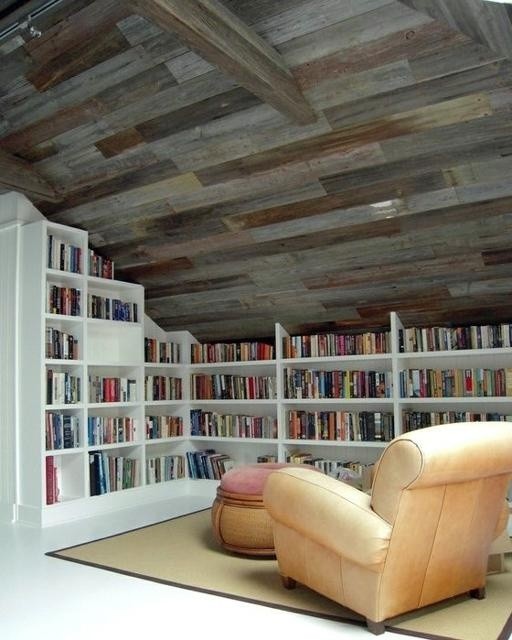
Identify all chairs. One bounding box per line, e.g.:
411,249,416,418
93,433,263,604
263,421,511,635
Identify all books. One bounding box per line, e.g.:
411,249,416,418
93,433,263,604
47,233,136,504
283,331,392,485
399,325,512,434
144,336,276,483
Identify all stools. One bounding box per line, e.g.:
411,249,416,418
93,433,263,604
210,462,318,560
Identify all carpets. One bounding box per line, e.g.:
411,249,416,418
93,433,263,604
42,506,511,640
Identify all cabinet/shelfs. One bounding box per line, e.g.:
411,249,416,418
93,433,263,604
1,192,511,555
